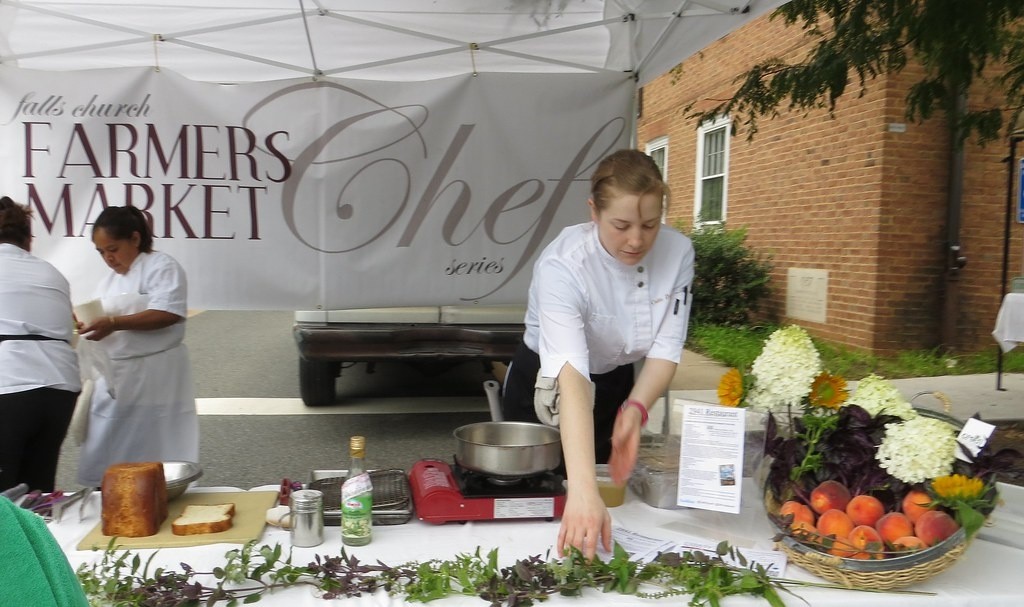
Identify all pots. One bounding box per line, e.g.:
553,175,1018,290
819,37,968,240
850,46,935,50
453,379,563,477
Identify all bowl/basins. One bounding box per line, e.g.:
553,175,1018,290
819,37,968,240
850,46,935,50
157,460,205,502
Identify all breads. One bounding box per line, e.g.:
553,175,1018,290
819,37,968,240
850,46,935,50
101,462,168,537
172,503,235,535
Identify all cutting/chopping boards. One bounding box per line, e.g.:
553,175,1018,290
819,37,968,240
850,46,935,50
76,492,279,552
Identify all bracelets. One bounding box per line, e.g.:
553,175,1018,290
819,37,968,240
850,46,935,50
618,400,648,426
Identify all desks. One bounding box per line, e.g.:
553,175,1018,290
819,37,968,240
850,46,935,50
990,293,1024,390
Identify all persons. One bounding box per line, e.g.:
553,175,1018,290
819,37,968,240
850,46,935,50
73,206,200,490
0,196,82,495
500,149,695,561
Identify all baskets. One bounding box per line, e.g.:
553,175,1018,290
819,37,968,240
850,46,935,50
758,393,997,592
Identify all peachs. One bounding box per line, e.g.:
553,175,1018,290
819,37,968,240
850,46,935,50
779,481,957,560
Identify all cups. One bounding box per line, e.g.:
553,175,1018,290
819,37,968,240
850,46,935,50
71,297,104,330
279,488,325,547
595,462,627,508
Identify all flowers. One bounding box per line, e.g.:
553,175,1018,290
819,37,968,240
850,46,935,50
912,474,998,540
790,372,849,479
717,370,756,409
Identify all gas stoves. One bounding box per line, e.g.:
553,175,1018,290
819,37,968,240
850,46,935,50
406,458,567,525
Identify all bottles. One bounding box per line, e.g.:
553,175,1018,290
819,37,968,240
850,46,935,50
339,434,374,547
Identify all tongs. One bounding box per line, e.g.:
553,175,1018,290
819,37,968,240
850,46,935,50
51,487,96,524
